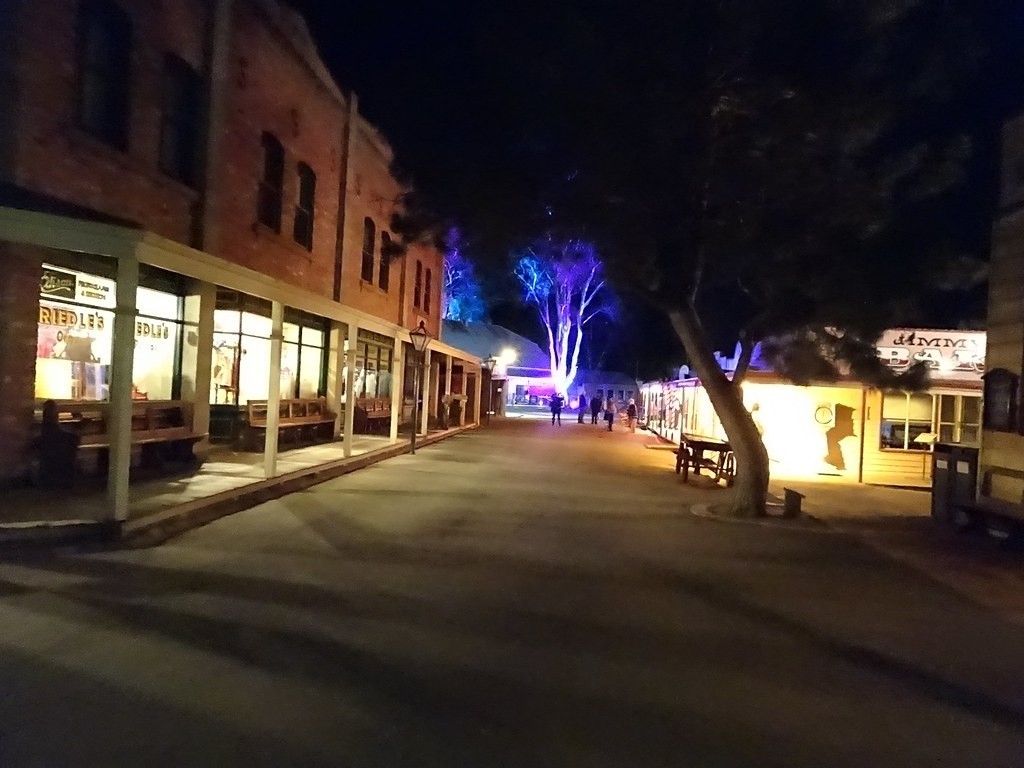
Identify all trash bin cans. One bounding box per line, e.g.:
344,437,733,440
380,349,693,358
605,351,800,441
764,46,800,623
928,443,979,520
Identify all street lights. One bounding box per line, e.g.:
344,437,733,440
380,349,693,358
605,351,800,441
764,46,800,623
407,320,434,455
483,353,497,424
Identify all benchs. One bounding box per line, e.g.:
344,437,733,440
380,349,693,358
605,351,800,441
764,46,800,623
242,396,334,445
41,400,209,477
355,398,391,433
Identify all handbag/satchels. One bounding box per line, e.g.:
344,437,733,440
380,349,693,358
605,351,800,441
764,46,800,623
604,411,613,420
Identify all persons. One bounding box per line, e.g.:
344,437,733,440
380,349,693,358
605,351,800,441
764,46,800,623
657,394,681,430
590,394,617,432
578,391,587,424
627,399,638,433
550,393,564,427
